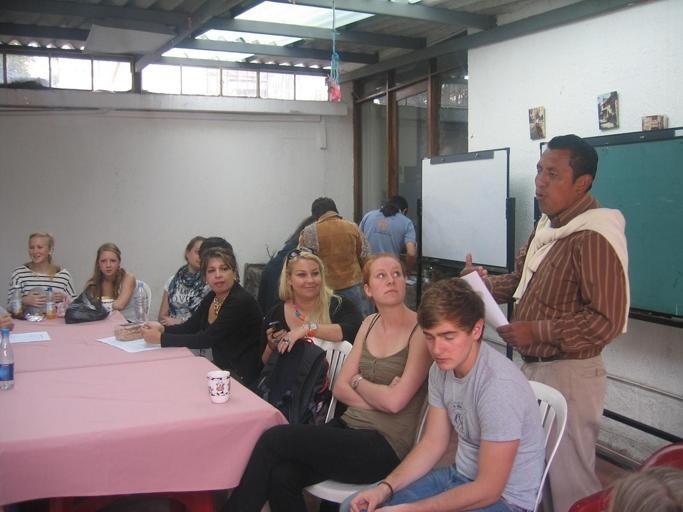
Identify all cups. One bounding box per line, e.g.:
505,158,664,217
102,299,112,313
206,370,232,404
47,287,56,320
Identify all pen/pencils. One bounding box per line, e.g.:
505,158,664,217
127,319,149,328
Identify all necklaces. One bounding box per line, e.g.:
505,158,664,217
103,278,113,282
213,296,225,318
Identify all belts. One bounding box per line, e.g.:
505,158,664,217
521,355,558,363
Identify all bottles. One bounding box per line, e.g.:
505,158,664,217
0,329,16,392
135,282,148,322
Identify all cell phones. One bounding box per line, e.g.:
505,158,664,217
269,321,282,333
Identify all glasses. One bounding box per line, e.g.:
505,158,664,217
286,247,313,265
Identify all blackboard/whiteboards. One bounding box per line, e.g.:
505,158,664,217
579,127,683,329
421,147,509,273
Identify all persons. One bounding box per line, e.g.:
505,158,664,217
609,466,682,512
459,133,631,511
141,236,266,394
0,306,14,332
158,236,213,326
83,243,137,326
232,196,544,511
7,232,77,315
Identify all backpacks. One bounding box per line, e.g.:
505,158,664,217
257,335,331,426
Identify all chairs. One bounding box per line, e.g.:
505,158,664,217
304,393,430,512
120,279,152,324
307,336,354,424
529,379,568,512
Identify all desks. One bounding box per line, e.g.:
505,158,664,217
0,311,194,371
0,354,290,511
